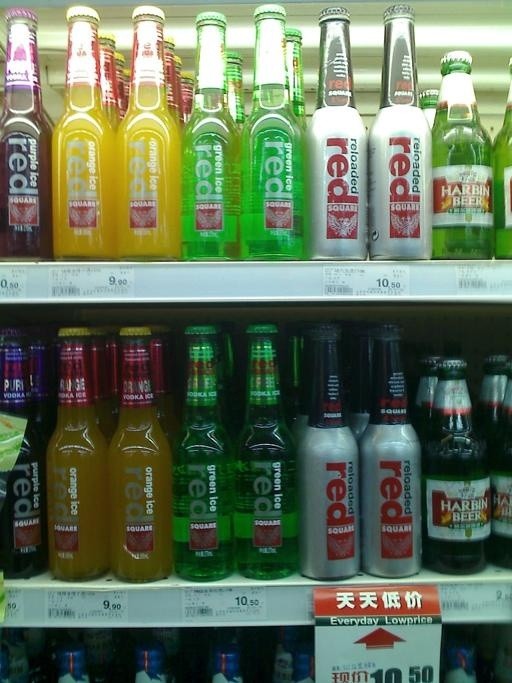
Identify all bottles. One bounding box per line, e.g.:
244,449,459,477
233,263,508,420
46,318,180,585
301,314,425,583
51,3,195,263
420,50,512,261
0,7,54,262
180,2,309,262
307,3,434,261
0,323,49,582
414,348,512,578
1,627,509,681
174,316,308,583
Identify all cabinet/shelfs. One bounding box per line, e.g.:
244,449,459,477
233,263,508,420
0,1,511,683
